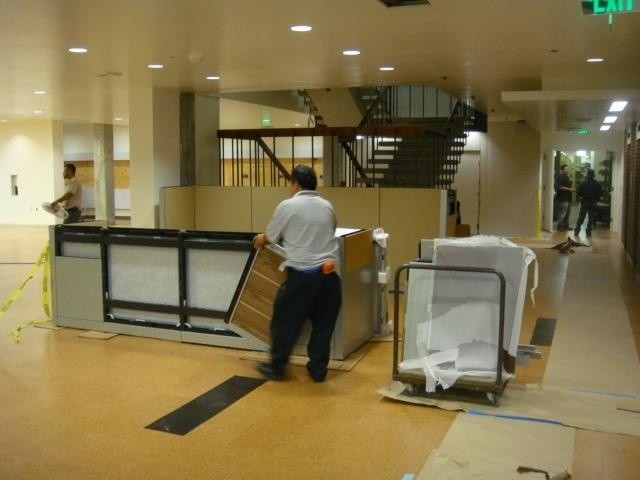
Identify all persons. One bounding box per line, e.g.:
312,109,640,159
249,163,344,384
50,163,84,225
554,164,577,232
573,168,603,238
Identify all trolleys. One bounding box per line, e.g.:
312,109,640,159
392,264,510,407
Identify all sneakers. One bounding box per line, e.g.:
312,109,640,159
257,363,287,380
558,226,591,236
